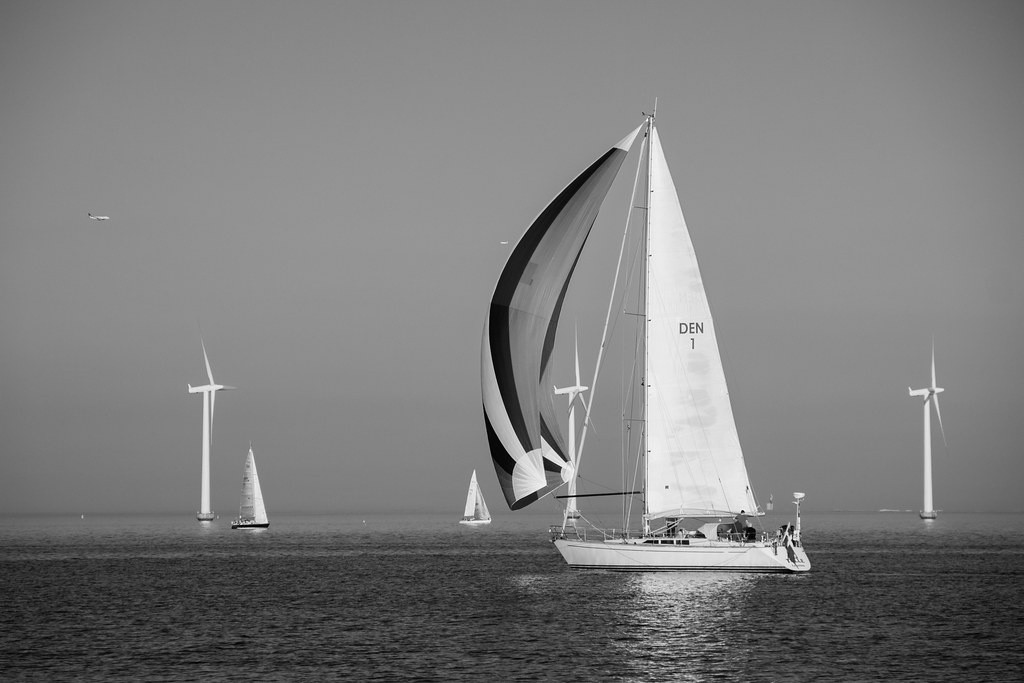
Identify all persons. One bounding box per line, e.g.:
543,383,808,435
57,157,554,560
734,510,751,538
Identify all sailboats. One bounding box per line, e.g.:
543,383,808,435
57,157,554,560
479,92,813,575
458,468,493,525
231,442,271,528
